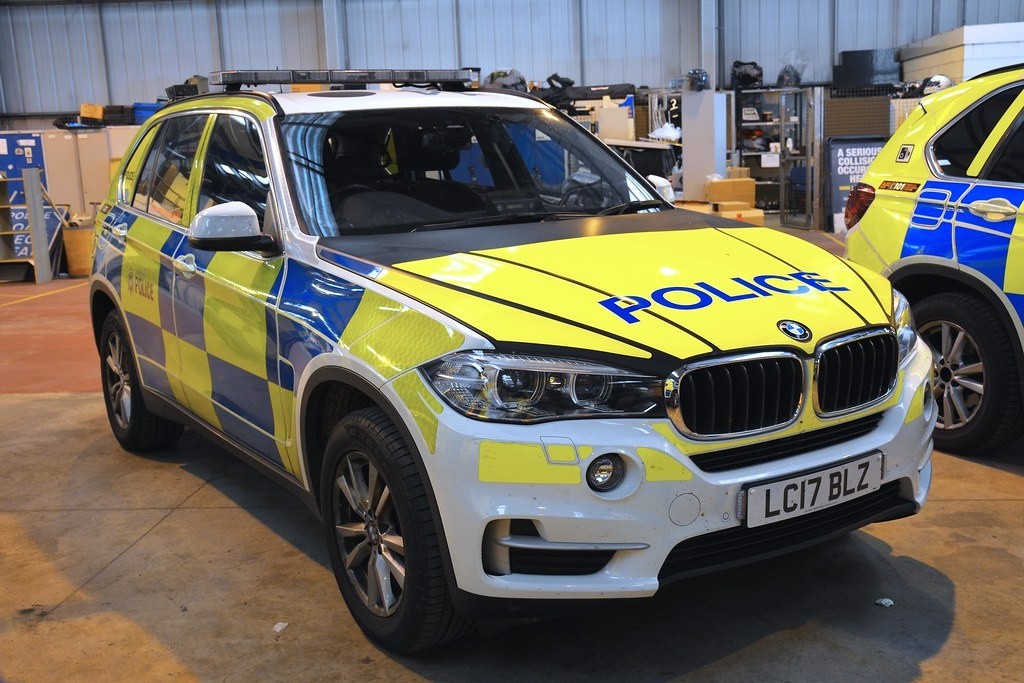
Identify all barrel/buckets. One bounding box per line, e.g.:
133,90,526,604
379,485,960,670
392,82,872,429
134,103,163,124
62,226,94,278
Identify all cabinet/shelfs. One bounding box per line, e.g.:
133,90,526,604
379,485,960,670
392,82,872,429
735,88,806,215
0,168,52,285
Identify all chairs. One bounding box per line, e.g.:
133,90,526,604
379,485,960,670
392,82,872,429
400,129,484,213
789,166,814,217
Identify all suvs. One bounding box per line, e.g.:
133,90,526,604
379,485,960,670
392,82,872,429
841,66,1024,454
87,66,938,658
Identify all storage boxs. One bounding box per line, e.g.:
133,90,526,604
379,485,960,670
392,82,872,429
54,82,199,128
674,167,764,226
